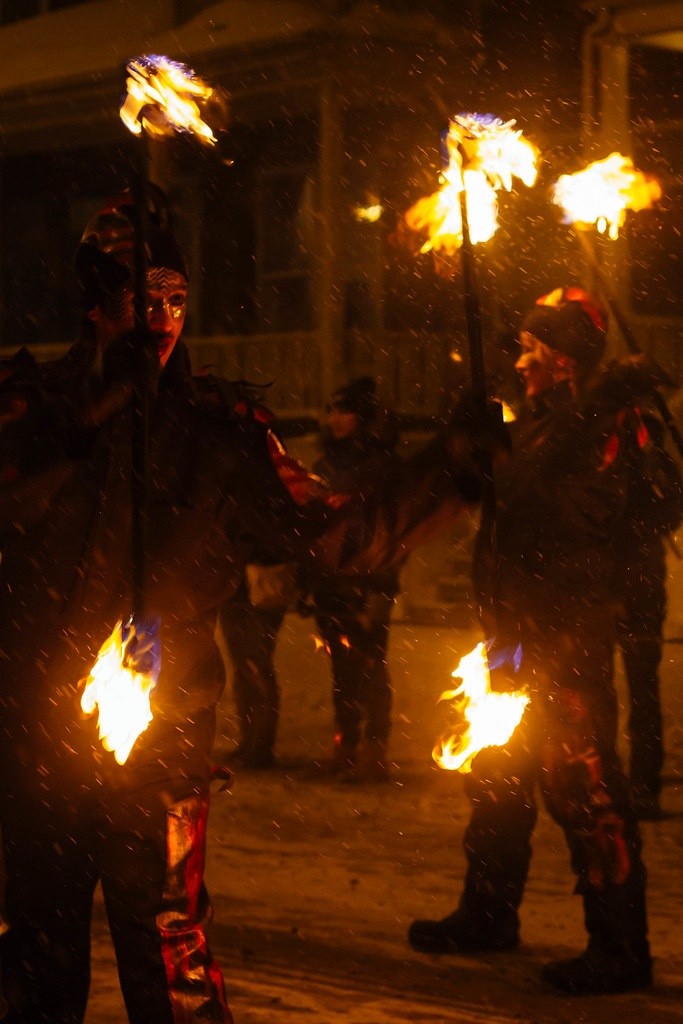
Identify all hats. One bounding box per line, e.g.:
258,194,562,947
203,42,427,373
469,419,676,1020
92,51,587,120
75,189,190,288
573,301,609,337
325,375,376,425
520,303,606,372
608,353,678,399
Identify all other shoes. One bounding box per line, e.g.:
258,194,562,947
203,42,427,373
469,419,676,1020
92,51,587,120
223,726,274,768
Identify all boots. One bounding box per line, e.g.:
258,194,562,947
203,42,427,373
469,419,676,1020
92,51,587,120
409,866,520,952
305,722,392,780
543,873,654,994
630,766,664,818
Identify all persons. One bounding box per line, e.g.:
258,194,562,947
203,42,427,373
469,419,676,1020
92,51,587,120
408,296,683,995
307,373,407,782
214,361,299,765
0,180,368,1024
